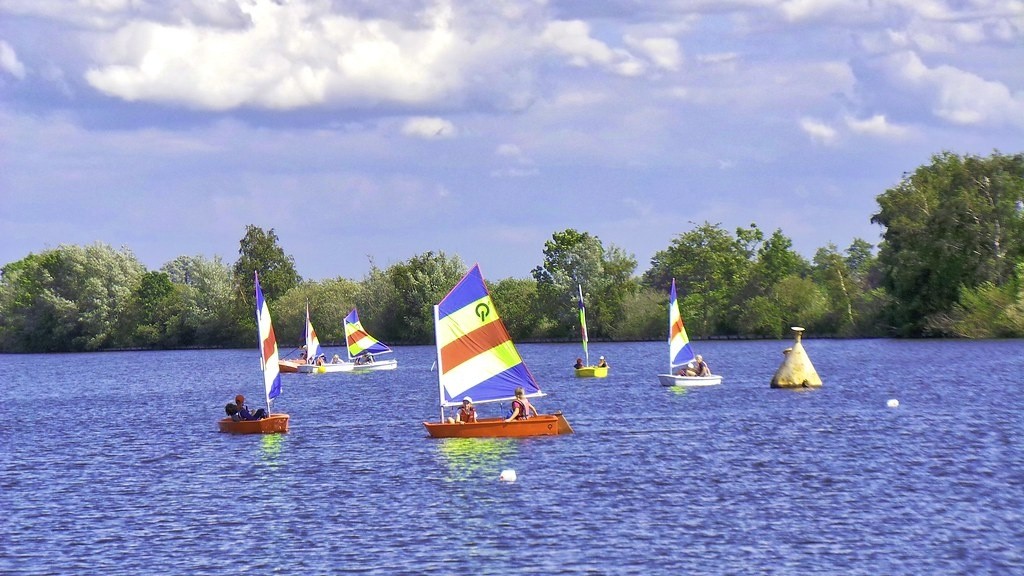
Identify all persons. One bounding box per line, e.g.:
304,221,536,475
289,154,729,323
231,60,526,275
598,355,608,367
317,357,324,365
331,354,343,364
355,350,375,364
505,388,538,422
447,396,477,423
687,355,710,377
573,359,584,368
301,351,305,359
226,395,266,420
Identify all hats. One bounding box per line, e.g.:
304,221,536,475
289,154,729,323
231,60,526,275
599,356,604,359
236,395,244,403
462,396,473,405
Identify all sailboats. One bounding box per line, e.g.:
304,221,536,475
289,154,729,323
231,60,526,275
422,261,578,439
216,269,291,436
574,282,606,378
278,297,355,374
343,305,398,371
657,277,724,389
